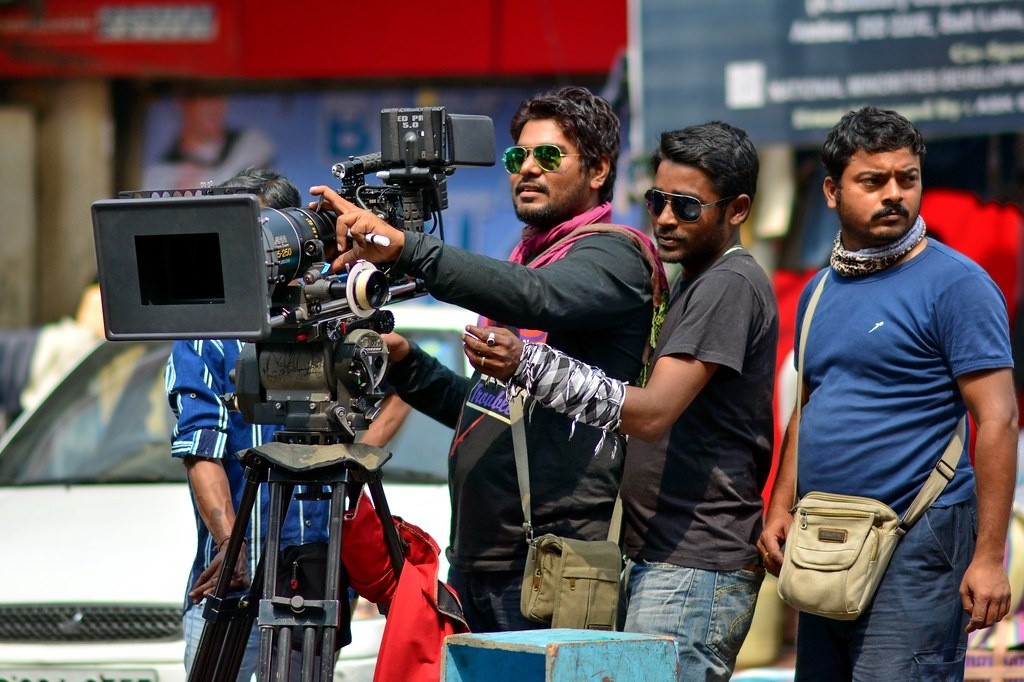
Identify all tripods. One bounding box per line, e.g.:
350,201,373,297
186,434,407,682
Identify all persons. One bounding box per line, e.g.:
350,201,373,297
461,123,779,682
310,86,671,633
167,166,414,682
756,105,1020,682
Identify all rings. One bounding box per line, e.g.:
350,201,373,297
763,552,769,562
487,333,495,345
481,356,485,365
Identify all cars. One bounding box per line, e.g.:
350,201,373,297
0,297,485,682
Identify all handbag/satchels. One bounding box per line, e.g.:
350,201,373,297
519,532,626,631
774,491,902,622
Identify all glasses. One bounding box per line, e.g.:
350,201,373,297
501,143,585,174
643,187,737,223
